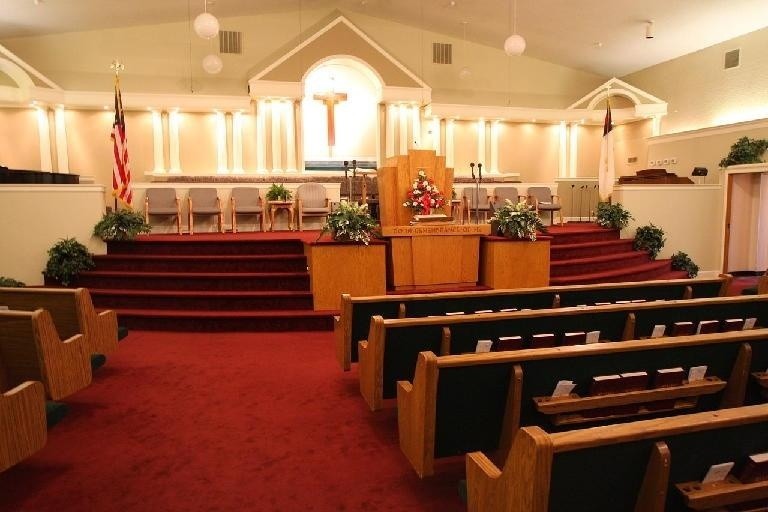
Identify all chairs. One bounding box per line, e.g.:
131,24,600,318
295,183,332,232
452,187,564,227
143,187,182,236
230,186,267,233
187,188,225,235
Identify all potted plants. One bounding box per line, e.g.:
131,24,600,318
266,182,293,201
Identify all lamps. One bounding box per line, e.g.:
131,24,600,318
645,20,655,40
504,0,526,56
203,38,223,75
193,0,219,39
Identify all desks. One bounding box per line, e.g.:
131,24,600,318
268,201,295,233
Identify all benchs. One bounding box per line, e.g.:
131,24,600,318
0,287,128,474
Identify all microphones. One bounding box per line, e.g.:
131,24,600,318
478,163,482,178
470,163,475,177
353,160,356,176
414,141,421,150
344,161,348,176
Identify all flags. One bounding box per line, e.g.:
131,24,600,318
597,104,615,201
108,81,134,209
